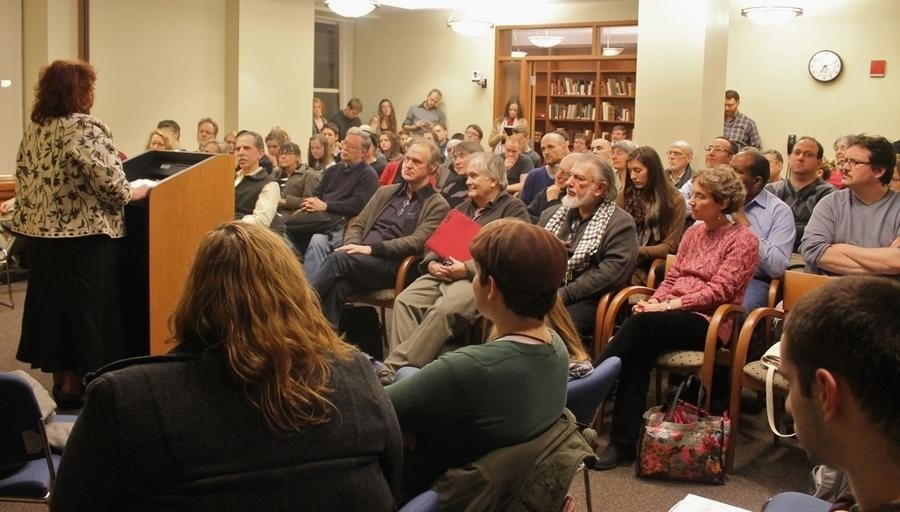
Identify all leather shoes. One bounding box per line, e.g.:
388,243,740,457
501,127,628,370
56,389,84,411
593,441,637,471
52,384,62,397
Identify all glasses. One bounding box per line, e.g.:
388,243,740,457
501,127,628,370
793,150,817,158
840,159,870,167
666,151,688,156
704,145,729,153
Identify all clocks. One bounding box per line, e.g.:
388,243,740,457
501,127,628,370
805,48,844,84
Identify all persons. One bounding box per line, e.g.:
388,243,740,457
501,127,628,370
140,87,899,474
386,214,569,491
760,272,900,512
11,57,155,413
50,220,406,511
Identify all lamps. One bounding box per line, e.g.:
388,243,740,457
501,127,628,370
738,2,807,29
511,29,527,58
323,0,383,21
526,28,566,49
602,27,625,57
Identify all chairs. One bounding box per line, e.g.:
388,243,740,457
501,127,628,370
0,375,83,511
635,256,782,345
394,419,588,512
726,270,874,475
761,489,834,511
1,217,21,312
586,277,630,431
560,354,622,431
591,255,747,434
345,255,421,353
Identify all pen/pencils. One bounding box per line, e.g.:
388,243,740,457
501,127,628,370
443,260,453,265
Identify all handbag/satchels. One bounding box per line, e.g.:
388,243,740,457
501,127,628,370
635,372,732,486
811,464,849,503
339,303,384,363
761,341,782,369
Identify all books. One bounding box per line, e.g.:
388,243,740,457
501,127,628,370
544,73,633,150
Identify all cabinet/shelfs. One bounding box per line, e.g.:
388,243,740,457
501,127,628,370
527,56,635,156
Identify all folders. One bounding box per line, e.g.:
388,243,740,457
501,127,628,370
424,209,483,263
